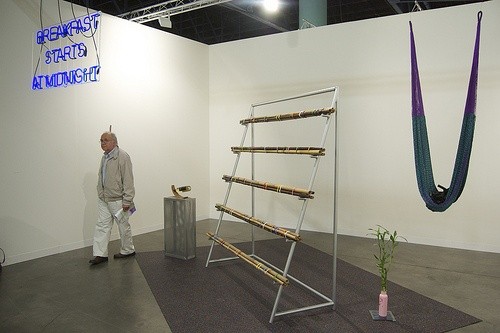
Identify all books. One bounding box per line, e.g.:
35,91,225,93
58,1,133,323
115,202,137,223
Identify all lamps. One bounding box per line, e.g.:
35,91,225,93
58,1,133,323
158,12,172,28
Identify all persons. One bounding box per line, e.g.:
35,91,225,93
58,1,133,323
88,132,135,266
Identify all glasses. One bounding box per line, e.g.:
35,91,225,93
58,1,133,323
100,138,114,142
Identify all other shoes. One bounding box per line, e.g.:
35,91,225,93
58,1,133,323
89,256,108,263
114,251,136,258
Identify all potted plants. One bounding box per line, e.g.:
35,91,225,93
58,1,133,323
366,225,408,317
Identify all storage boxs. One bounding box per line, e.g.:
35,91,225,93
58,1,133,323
163,196,196,261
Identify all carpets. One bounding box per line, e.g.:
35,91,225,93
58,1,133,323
135,238,482,333
